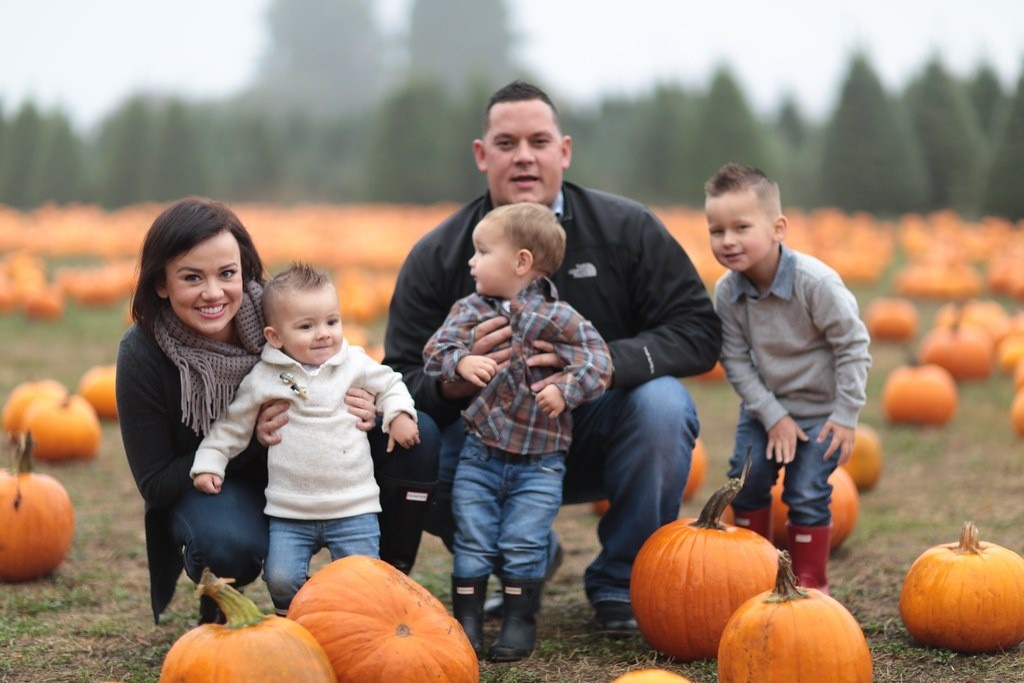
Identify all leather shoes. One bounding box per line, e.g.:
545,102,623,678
197,589,245,626
591,600,642,637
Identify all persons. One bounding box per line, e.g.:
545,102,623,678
420,200,614,660
705,158,873,598
115,197,443,634
380,81,725,642
187,263,425,620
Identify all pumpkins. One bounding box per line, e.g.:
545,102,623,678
0,194,999,683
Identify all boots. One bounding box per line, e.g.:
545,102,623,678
732,504,774,544
449,570,490,660
487,573,545,663
780,519,835,599
